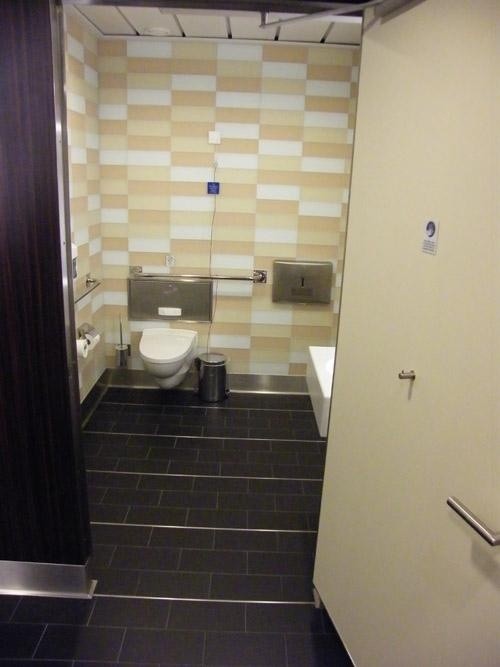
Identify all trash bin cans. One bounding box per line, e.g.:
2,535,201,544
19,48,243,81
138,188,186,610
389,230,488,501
195,352,227,403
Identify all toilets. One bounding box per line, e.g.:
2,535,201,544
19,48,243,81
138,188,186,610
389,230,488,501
137,328,197,390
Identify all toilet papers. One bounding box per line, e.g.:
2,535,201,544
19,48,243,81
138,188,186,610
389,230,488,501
76,339,89,358
79,331,100,351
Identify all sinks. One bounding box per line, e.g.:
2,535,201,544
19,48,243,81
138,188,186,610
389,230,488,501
305,345,336,438
